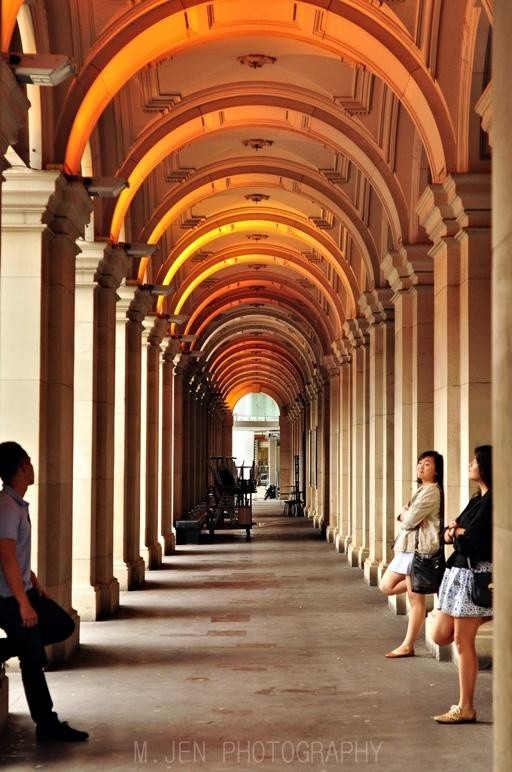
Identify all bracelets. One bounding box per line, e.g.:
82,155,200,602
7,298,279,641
448,526,456,539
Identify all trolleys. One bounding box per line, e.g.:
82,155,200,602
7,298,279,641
205,485,258,539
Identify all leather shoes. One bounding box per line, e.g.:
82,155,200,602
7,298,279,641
434,704,476,723
385,648,414,658
36,721,89,744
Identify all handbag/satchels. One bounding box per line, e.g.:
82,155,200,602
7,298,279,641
471,571,492,609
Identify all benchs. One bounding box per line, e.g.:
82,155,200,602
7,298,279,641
175,502,207,544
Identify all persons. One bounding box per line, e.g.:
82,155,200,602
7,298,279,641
2,438,94,747
428,445,493,725
376,450,447,659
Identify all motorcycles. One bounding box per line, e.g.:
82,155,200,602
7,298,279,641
263,483,280,500
